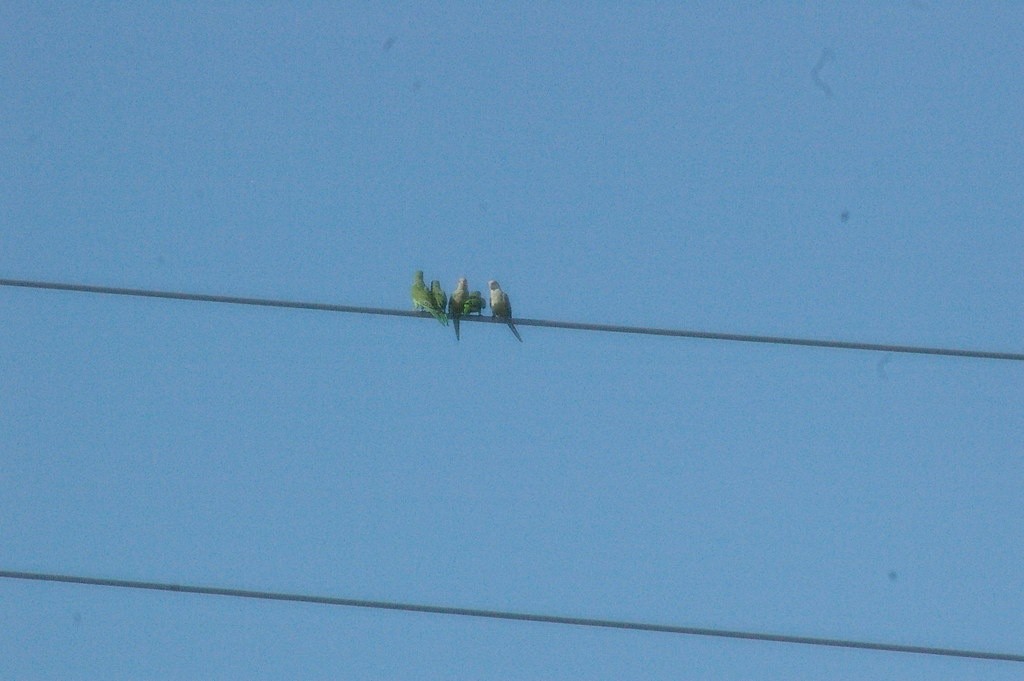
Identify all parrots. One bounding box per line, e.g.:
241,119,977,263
412,269,526,346
